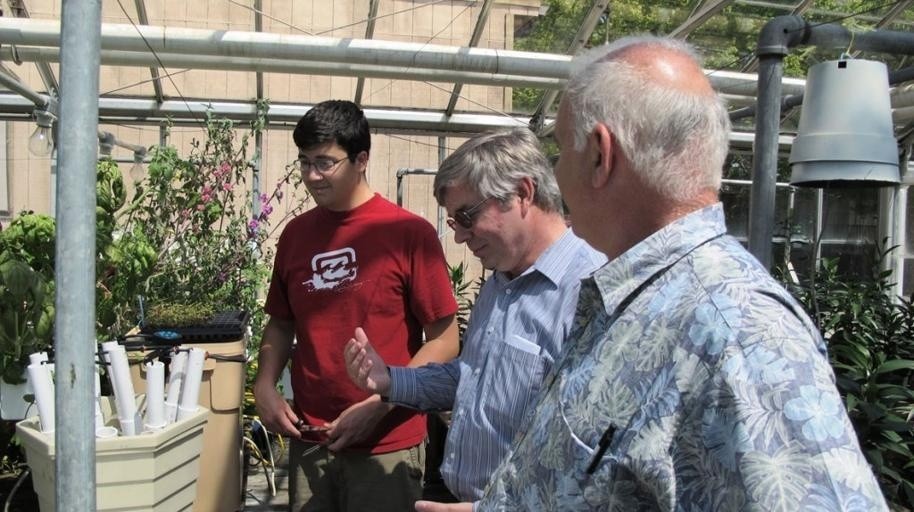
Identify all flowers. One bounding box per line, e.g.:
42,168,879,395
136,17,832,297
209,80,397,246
159,164,283,286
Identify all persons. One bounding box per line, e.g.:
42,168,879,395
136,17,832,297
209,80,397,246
254,98,461,510
474,33,895,509
343,125,611,512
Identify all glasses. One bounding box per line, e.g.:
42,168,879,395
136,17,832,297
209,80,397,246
293,157,349,172
446,196,493,231
